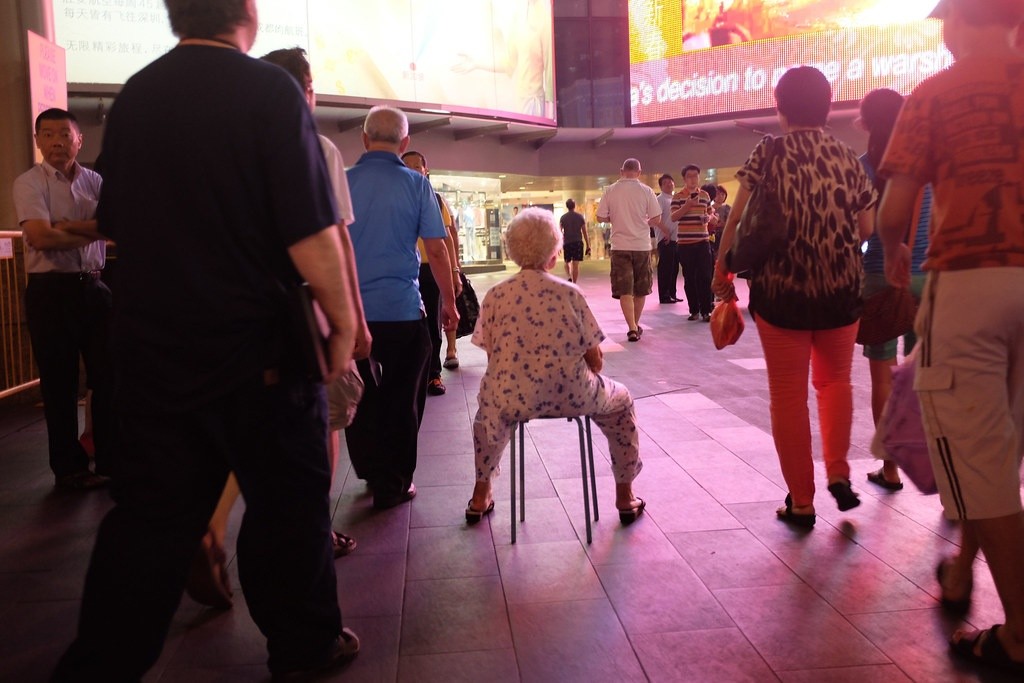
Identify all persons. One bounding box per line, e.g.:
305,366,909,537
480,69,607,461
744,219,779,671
877,1,1024,683
702,184,731,309
13,108,112,491
449,0,553,120
401,152,463,396
671,164,715,322
43,0,359,682
344,105,461,511
857,88,933,492
654,175,684,303
513,207,518,215
559,199,591,284
710,66,878,527
594,158,662,342
465,206,646,525
186,48,373,612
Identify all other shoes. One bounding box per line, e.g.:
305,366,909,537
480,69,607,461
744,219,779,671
429,378,446,395
53,475,83,508
703,314,711,322
688,312,699,320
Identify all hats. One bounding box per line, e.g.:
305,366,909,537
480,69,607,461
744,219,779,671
928,0,1024,19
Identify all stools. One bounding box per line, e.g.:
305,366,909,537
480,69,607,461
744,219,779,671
511,413,598,546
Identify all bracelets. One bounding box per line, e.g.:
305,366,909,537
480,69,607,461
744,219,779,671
453,267,460,273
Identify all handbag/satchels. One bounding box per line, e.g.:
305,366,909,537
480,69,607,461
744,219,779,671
871,343,940,497
709,272,745,350
854,279,919,346
455,272,482,339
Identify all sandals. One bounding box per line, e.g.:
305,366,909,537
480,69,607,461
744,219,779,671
330,530,357,558
627,330,640,342
191,527,233,608
273,625,360,683
639,327,643,335
464,496,495,523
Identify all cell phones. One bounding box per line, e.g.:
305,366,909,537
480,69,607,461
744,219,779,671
691,192,698,199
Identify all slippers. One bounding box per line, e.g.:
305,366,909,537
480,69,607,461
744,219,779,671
868,467,904,490
776,494,816,528
948,624,1024,676
827,477,861,512
935,554,974,615
444,357,459,368
619,497,646,522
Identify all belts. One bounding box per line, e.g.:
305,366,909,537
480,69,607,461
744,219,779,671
27,271,104,283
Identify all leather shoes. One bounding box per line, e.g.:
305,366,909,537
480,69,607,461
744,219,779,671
372,483,416,510
660,297,683,303
